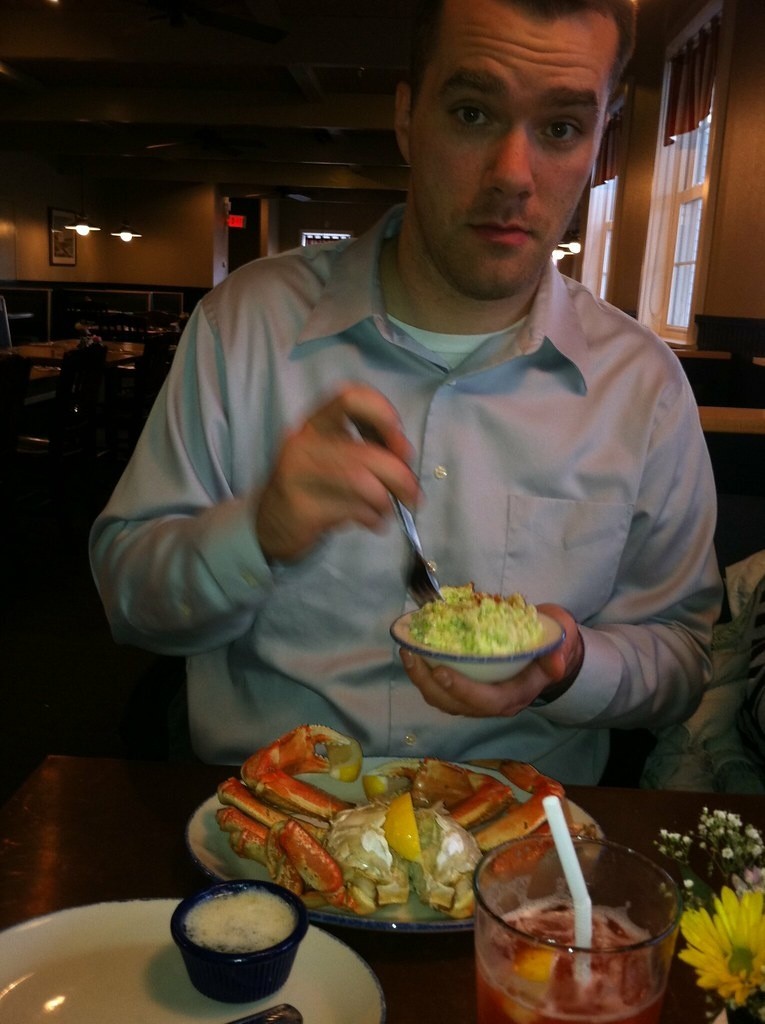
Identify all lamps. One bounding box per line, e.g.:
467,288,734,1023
108,163,143,241
64,162,101,237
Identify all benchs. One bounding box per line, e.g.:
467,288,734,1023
152,293,183,326
0,287,51,343
53,288,152,334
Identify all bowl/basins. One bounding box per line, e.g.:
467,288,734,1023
390,609,566,684
170,878,309,1004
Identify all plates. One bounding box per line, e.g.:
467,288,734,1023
184,757,606,933
0,898,385,1024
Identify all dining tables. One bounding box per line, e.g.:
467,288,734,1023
0,754,765,1024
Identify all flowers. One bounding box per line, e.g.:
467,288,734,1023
653,806,765,1024
78,335,103,348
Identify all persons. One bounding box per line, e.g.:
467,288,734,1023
89,0,723,787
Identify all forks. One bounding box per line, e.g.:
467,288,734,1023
348,413,445,609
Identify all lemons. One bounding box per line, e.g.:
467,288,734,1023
494,941,561,1024
325,735,362,782
363,760,422,801
385,791,423,863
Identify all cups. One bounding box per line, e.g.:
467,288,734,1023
473,836,683,1024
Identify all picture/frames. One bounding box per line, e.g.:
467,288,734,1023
48,206,77,267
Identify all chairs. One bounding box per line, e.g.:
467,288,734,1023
0,311,181,509
0,296,13,349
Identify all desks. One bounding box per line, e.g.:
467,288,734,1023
7,313,34,319
6,337,177,466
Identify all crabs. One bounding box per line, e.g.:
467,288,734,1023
214,721,600,921
